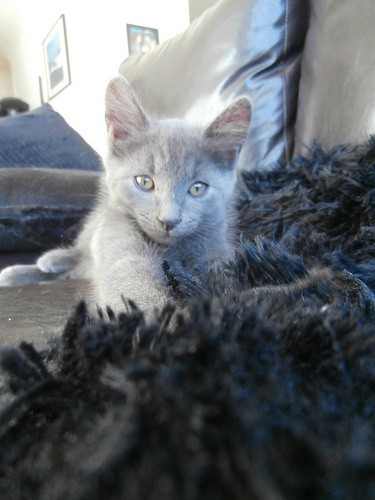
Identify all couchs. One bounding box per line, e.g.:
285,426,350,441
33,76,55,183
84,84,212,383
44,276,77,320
0,0,375,500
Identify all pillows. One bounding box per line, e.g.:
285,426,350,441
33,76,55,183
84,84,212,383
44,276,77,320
0,102,106,250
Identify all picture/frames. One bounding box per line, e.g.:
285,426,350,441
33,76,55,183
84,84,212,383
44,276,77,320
126,24,160,58
40,14,71,100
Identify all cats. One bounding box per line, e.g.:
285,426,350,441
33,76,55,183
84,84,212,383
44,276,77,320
0,74,254,334
